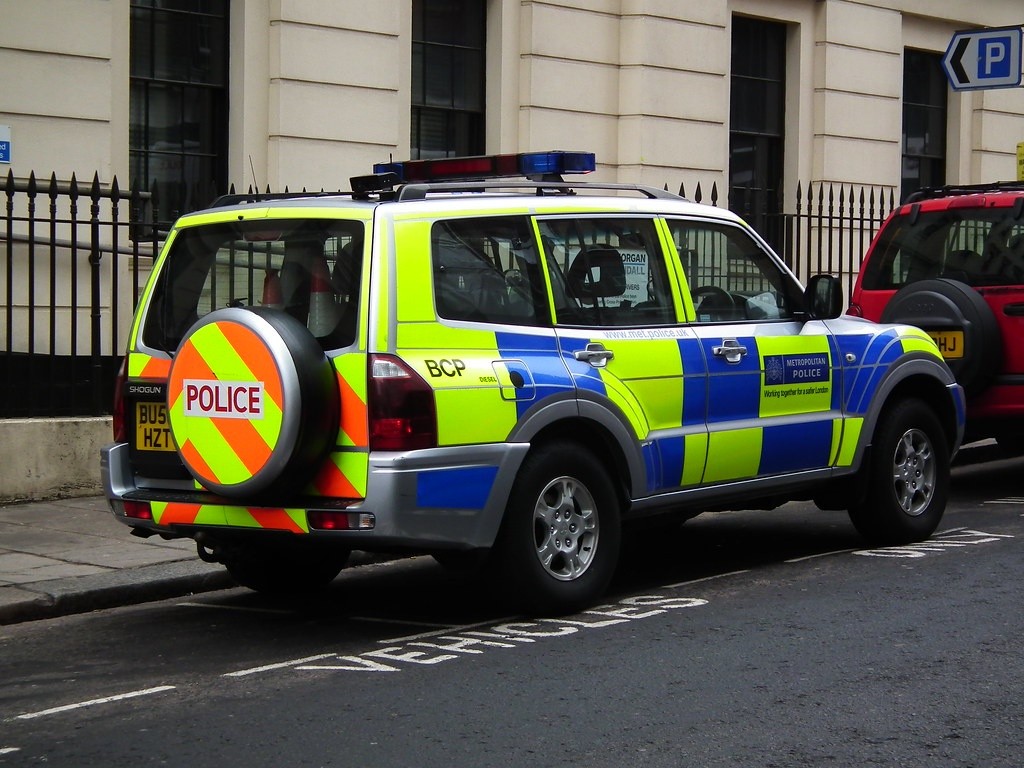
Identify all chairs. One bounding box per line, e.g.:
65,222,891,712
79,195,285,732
564,243,664,326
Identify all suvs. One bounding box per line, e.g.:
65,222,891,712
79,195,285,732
99,151,969,618
845,181,1024,449
